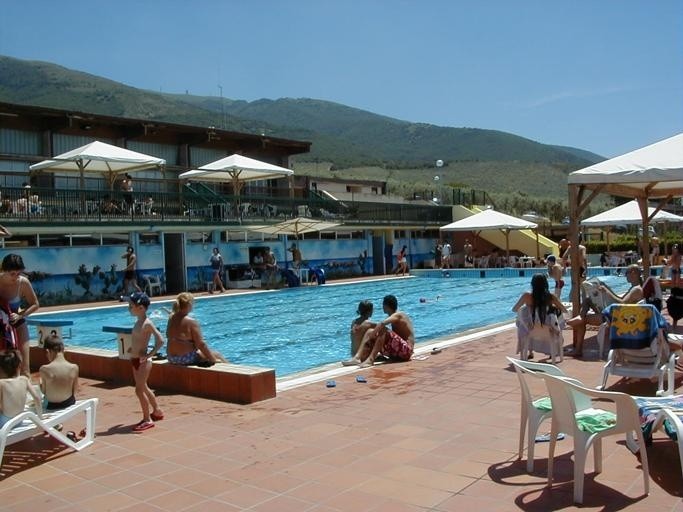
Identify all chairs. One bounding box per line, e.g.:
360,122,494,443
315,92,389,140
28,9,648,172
504,285,683,504
143,274,160,294
0,392,99,471
473,254,535,268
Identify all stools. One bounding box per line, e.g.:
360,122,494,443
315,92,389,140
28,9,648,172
203,282,216,291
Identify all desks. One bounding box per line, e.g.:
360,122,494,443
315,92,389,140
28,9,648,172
289,269,309,284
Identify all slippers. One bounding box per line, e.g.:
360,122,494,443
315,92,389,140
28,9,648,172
55,424,96,442
534,432,564,441
152,352,168,360
431,348,442,354
356,375,368,382
326,380,336,386
133,413,164,430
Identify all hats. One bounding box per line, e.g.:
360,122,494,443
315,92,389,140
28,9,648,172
120,292,150,305
548,256,556,263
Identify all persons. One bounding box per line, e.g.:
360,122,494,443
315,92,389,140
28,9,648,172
0,350,44,428
207,246,227,296
120,290,165,433
252,243,302,287
394,244,409,278
148,304,174,321
416,293,443,304
167,291,228,367
117,245,143,302
38,329,79,411
539,233,682,314
0,223,12,239
0,254,42,384
349,296,376,360
434,237,505,269
0,172,276,218
339,294,415,369
566,264,645,358
512,273,570,317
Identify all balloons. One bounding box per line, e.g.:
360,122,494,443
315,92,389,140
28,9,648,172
433,176,440,181
435,159,443,167
